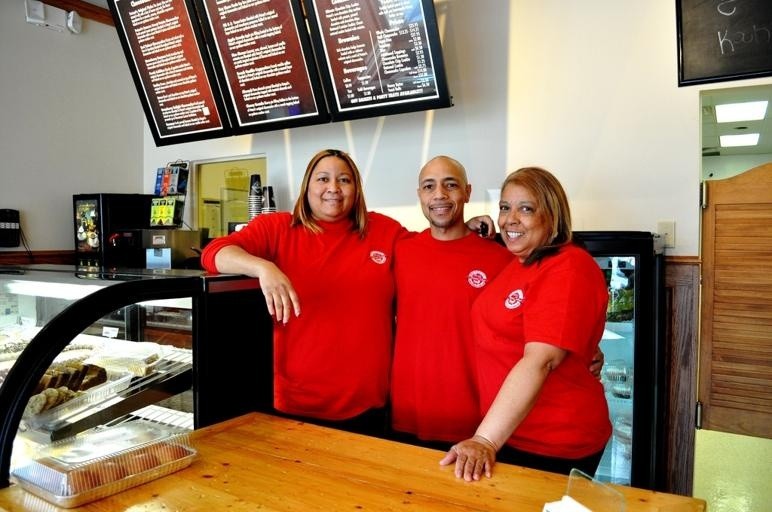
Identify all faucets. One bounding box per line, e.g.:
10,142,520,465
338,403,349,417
190,247,202,257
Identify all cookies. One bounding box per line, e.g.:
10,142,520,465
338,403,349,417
22,386,86,420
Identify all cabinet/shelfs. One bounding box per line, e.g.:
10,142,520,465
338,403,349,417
568,231,671,494
73,192,164,267
0,264,275,487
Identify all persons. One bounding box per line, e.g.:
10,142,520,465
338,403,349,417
438,167,613,482
388,156,605,453
200,150,497,442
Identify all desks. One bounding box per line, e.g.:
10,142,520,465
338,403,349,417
0,409,707,512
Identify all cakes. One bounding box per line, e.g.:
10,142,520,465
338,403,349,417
34,363,107,391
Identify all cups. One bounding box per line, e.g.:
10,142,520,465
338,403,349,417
261,185,277,213
248,174,261,221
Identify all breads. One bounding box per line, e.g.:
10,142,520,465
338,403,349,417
157,444,188,466
66,468,99,496
97,461,126,485
124,452,161,476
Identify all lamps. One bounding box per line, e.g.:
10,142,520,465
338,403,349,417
25,0,83,34
